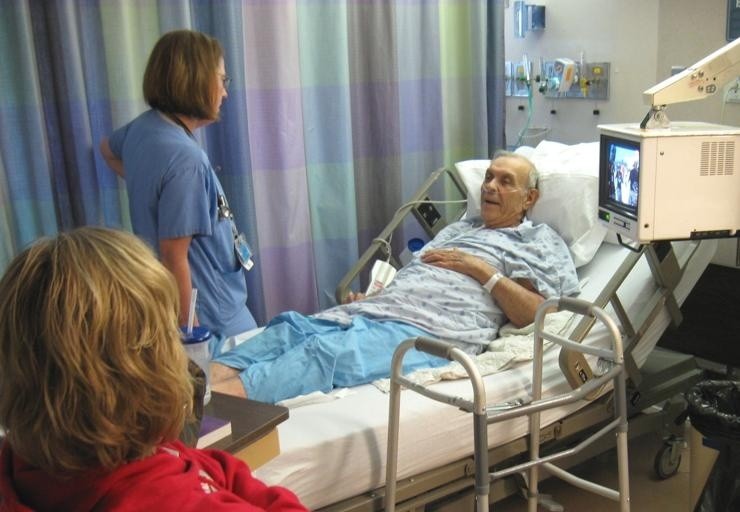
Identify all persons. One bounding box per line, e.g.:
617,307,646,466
198,147,585,402
2,223,316,512
100,29,260,339
605,161,641,209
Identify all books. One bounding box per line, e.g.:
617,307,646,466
194,415,233,449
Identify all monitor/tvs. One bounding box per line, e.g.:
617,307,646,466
593,122,740,246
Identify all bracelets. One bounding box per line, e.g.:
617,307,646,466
482,271,504,294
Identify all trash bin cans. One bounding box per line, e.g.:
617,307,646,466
689,380,739,512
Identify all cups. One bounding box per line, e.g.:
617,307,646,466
179,326,213,408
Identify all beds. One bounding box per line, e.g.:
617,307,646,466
186,158,715,512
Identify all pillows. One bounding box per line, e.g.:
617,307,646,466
495,144,604,268
